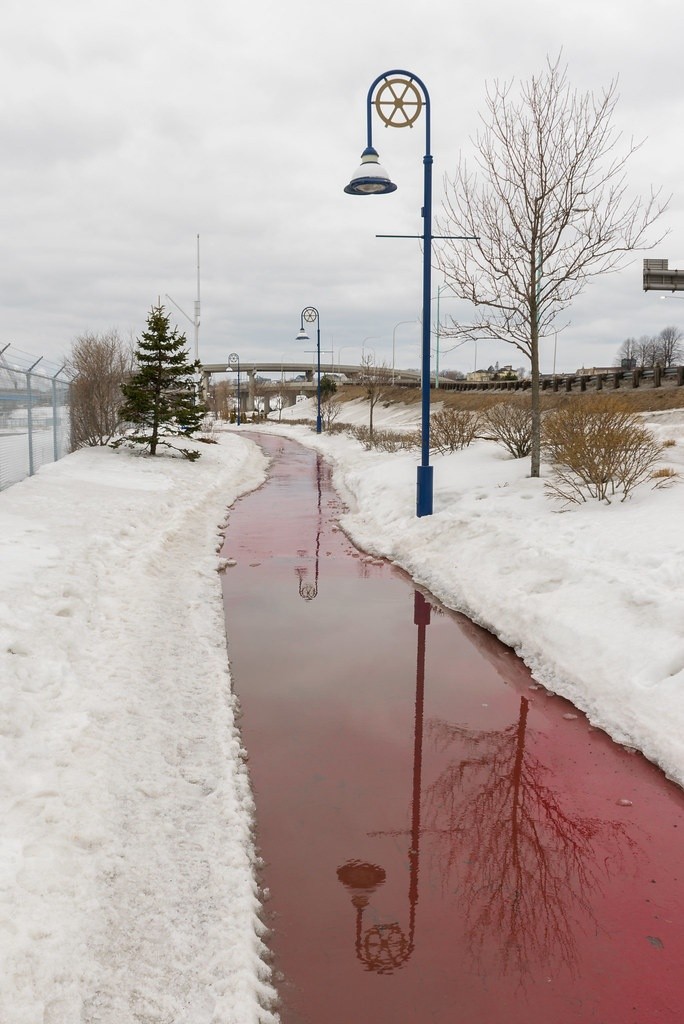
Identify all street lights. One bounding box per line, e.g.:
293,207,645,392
226,353,240,426
295,307,321,434
435,282,472,388
392,321,418,385
342,68,433,518
361,336,380,387
